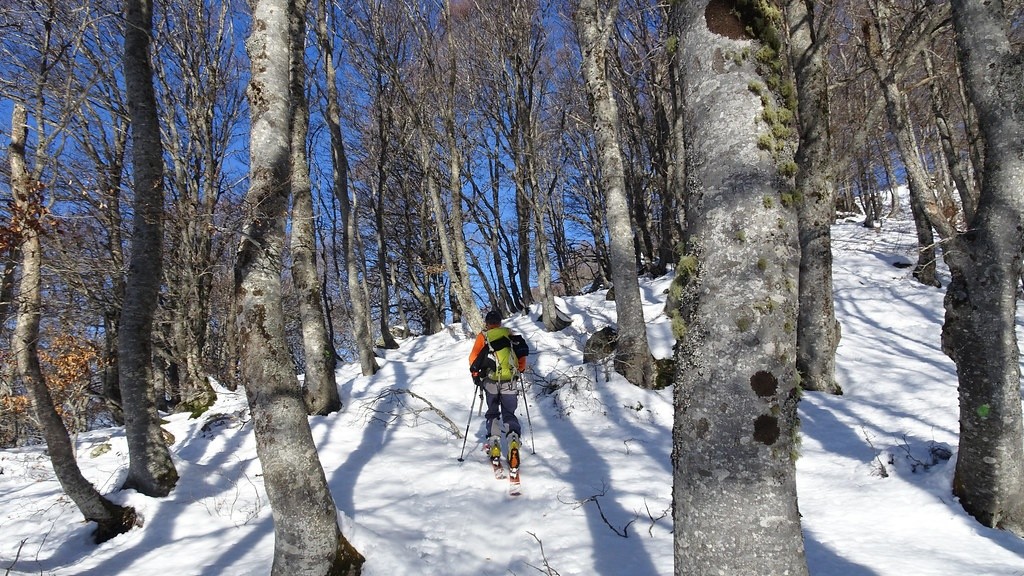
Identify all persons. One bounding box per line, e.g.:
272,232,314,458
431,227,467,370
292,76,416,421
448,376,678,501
469,311,529,473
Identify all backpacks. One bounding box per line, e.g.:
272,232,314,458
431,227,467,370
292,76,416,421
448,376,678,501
480,327,519,380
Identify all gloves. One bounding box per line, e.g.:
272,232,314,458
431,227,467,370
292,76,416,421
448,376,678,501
473,373,482,386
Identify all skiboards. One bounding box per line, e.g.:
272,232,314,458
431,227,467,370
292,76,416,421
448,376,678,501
482,439,522,496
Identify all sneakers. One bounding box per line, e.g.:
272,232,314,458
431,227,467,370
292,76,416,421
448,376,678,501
508,441,520,473
490,446,500,469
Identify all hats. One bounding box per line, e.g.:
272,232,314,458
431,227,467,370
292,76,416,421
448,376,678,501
486,311,501,322
